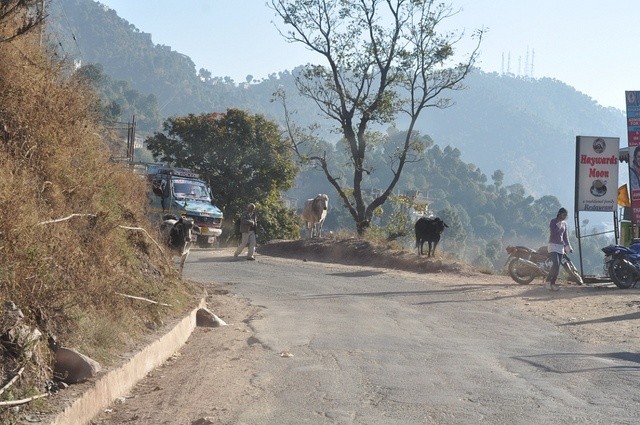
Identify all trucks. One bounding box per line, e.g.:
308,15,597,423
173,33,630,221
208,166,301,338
149,164,224,248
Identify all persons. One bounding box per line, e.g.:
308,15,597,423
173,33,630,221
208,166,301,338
544,206,573,292
233,202,259,261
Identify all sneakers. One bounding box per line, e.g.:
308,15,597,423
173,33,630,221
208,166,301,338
233,252,239,260
544,280,552,290
551,284,559,291
247,255,254,259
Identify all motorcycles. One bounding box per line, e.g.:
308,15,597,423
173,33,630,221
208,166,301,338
503,246,584,287
602,243,637,288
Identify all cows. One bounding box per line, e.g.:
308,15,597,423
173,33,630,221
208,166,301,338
302,194,329,239
236,213,259,247
159,216,201,276
415,216,449,258
